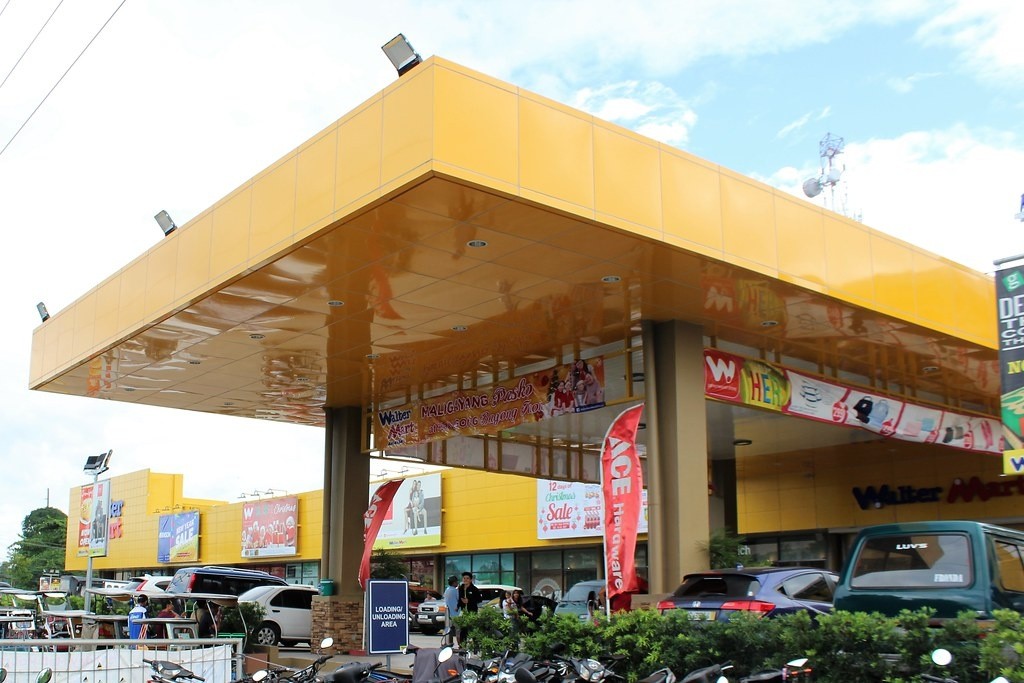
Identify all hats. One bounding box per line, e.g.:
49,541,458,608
138,594,149,606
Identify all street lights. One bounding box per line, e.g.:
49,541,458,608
84,450,111,614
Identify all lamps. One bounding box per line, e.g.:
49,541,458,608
380,31,424,75
36,302,52,324
153,209,179,239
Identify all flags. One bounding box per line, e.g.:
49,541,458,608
357,479,403,591
600,404,645,600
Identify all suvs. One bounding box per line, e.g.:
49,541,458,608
417,585,524,636
164,567,289,621
125,573,174,595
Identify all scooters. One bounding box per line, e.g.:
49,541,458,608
5,637,1012,683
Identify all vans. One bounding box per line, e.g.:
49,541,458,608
835,521,1024,641
555,580,605,625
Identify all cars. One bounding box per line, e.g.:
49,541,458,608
407,587,443,633
655,567,840,625
237,584,319,648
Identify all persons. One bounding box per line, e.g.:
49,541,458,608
205,602,219,619
583,372,601,406
155,599,182,638
454,572,482,653
573,380,587,409
411,480,428,535
511,589,533,620
562,380,576,415
190,599,222,640
404,481,418,533
412,491,422,522
126,594,151,650
502,592,519,625
571,360,600,391
241,516,295,549
550,381,566,418
586,591,597,623
443,576,465,654
